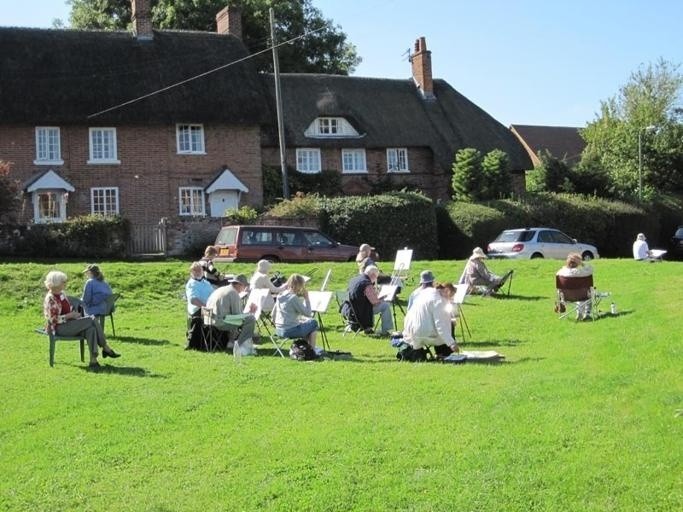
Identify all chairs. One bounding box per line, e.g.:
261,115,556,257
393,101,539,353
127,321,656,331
335,290,365,336
197,307,224,351
556,275,597,324
95,293,116,336
33,297,84,365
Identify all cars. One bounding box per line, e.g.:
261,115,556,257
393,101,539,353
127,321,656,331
483,227,600,263
671,224,682,261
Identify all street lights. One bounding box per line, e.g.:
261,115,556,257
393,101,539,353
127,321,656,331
637,125,663,199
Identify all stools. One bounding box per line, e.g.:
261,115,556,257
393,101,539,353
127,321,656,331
271,333,292,358
422,345,434,362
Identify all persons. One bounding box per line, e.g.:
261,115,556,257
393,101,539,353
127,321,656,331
72,264,114,318
43,270,121,373
408,269,435,311
673,225,683,246
249,259,288,326
556,253,593,320
270,274,323,355
403,282,459,363
347,265,393,338
356,244,402,305
464,247,501,296
185,246,257,351
633,232,655,263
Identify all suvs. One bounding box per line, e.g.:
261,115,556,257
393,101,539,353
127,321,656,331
209,223,380,265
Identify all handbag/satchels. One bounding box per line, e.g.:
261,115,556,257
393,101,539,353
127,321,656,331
289,339,315,361
554,303,566,313
396,344,426,362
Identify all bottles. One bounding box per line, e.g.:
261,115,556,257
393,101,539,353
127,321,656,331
611,301,616,314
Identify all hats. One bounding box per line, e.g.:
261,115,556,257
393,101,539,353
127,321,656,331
470,247,488,261
360,244,375,251
228,274,250,285
420,270,435,284
257,259,273,273
82,265,100,273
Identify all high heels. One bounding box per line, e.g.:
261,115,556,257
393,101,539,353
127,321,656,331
103,349,121,358
89,363,102,368
